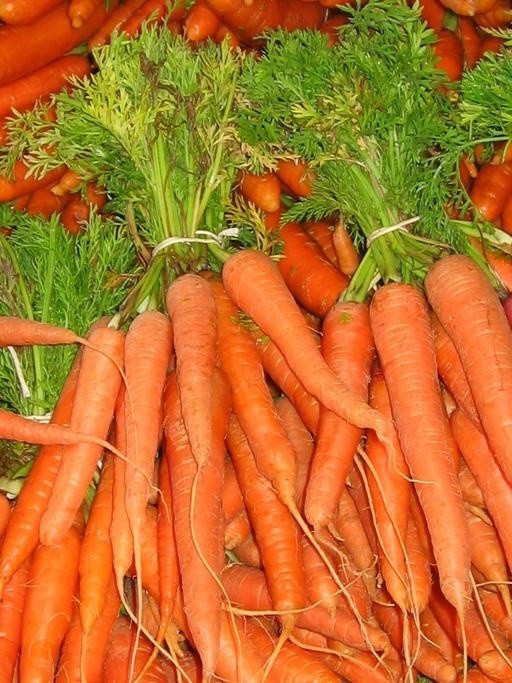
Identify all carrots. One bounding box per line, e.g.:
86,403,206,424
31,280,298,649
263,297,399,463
0,0,512,683
